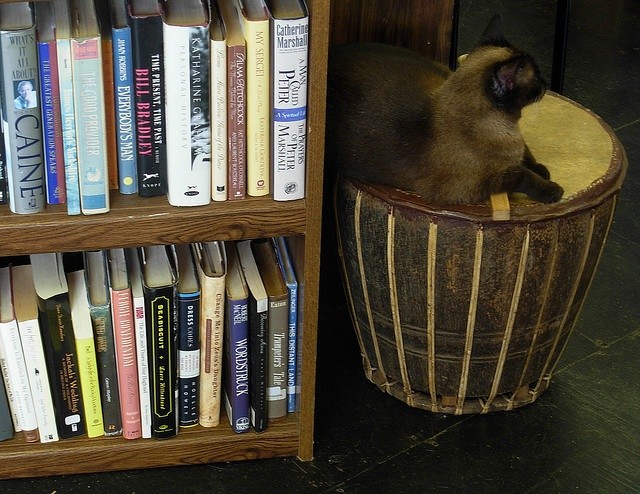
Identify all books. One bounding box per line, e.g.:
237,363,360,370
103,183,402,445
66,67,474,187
0,1,307,214
0,238,299,443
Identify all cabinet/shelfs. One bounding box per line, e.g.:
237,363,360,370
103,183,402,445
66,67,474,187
0,0,329,481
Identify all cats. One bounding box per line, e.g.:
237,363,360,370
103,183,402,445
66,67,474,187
328,16,564,205
